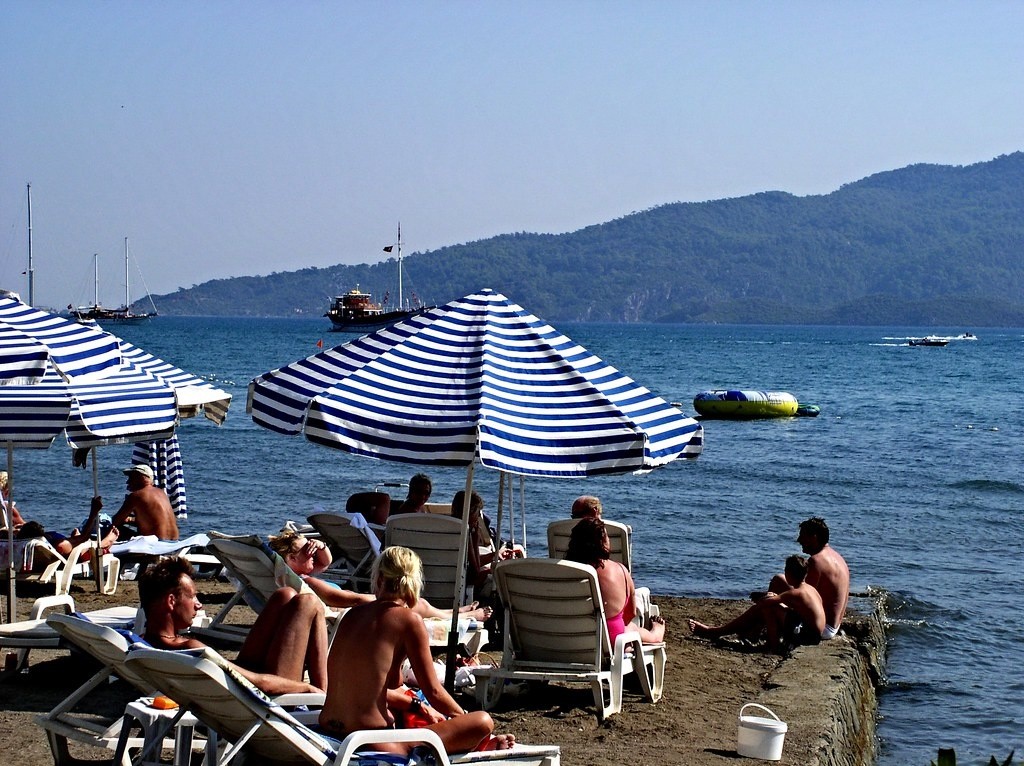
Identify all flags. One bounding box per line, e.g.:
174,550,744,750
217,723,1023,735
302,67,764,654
67,304,72,309
383,246,393,252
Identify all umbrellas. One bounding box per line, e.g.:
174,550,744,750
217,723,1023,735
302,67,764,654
0,291,232,624
131,429,187,520
247,285,706,696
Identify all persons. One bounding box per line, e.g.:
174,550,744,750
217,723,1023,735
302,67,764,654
137,556,328,713
0,471,119,564
318,546,516,755
570,496,602,519
563,518,665,652
111,464,179,542
450,490,513,567
269,530,493,622
688,515,850,647
397,473,432,514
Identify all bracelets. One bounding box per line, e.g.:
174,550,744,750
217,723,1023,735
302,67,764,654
407,698,421,713
318,542,326,551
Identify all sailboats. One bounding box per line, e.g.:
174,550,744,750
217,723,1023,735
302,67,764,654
66,236,161,326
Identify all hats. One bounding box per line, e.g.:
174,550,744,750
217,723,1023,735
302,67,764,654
124,464,153,482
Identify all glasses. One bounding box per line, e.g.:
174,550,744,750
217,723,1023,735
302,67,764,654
412,490,431,496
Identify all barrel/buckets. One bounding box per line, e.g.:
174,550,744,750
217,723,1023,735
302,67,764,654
736,703,789,761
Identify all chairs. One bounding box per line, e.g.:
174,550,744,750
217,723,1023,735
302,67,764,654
1,498,665,766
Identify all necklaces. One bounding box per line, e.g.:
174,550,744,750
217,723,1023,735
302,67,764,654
159,634,183,639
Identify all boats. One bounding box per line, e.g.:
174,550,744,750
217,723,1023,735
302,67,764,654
322,220,438,335
908,337,950,346
